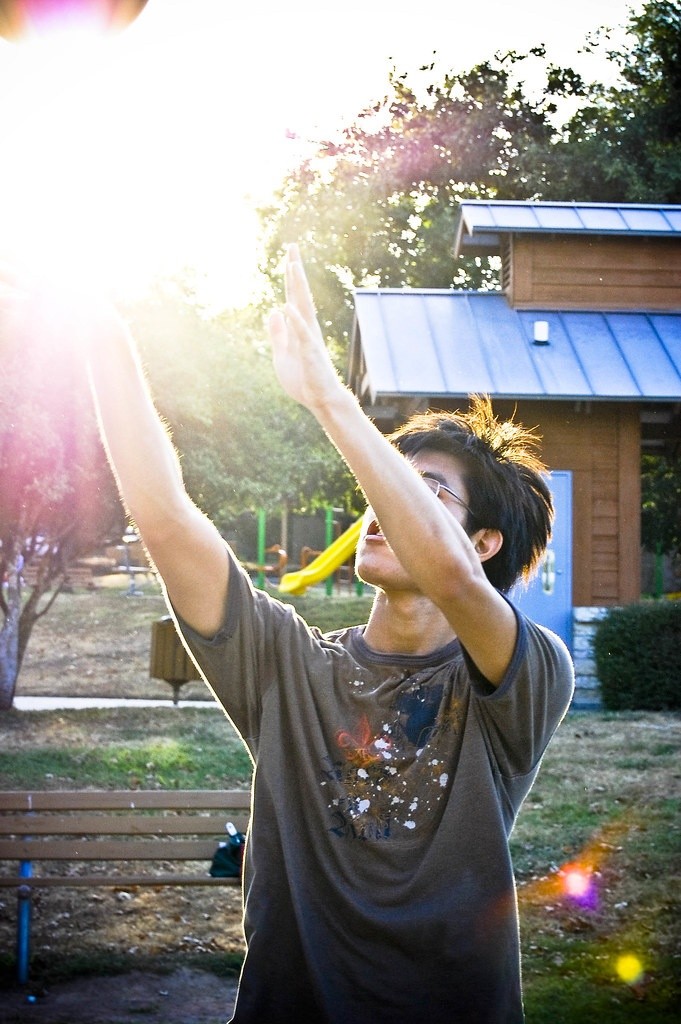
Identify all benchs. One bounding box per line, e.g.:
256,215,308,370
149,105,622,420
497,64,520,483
23,567,96,593
0,792,252,981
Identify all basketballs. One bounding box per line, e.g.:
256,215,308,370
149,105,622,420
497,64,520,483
0,0,149,45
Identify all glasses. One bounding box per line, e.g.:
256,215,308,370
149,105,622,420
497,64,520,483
423,477,476,517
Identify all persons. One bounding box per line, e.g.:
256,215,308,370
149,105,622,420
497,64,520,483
80,240,578,1024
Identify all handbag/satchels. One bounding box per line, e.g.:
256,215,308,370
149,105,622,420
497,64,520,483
210,833,247,878
